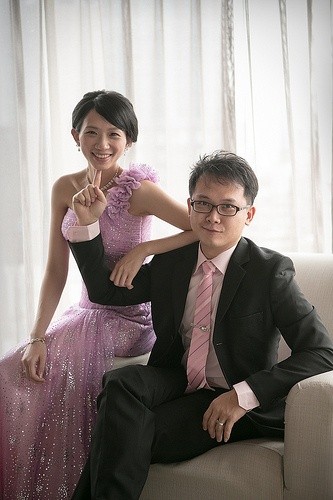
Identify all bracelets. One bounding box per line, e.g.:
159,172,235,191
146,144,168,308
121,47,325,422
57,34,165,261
29,337,45,345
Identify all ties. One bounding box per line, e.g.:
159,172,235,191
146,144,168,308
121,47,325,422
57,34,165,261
185,260,219,392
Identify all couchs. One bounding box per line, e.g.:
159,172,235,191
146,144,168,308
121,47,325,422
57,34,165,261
102,253,333,500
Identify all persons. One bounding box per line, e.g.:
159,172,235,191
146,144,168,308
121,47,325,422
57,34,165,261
0,90,204,500
68,150,333,500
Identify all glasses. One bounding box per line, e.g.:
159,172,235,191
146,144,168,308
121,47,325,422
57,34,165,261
190,198,248,217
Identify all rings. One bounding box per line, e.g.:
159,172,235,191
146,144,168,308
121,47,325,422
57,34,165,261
216,419,223,425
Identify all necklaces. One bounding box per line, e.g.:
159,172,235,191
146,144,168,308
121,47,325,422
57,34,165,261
100,165,119,192
